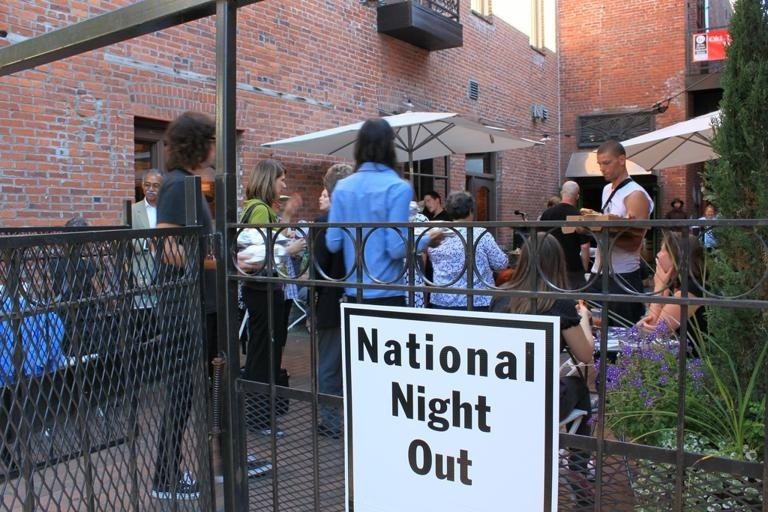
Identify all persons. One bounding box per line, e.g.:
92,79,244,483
58,217,116,358
537,194,560,221
152,109,273,499
318,186,331,212
634,230,708,358
666,197,688,220
698,205,722,249
239,158,308,436
323,120,447,309
426,190,510,315
490,230,596,503
313,164,354,438
422,190,451,219
586,142,654,326
125,168,165,376
284,219,311,333
540,181,589,293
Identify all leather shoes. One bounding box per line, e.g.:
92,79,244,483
312,424,343,439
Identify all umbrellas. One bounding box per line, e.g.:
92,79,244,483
259,110,549,181
619,109,725,172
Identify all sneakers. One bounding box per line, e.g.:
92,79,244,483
154,472,201,501
214,457,274,482
566,472,597,505
255,427,285,437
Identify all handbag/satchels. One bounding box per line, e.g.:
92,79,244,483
239,254,297,305
